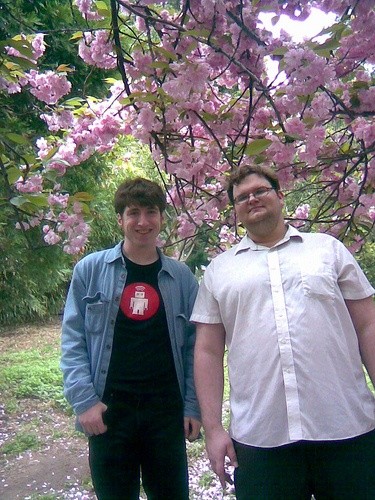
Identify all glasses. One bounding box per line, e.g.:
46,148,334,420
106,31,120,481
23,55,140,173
232,186,277,206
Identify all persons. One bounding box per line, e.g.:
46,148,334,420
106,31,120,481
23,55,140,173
189,166,375,500
60,177,202,500
